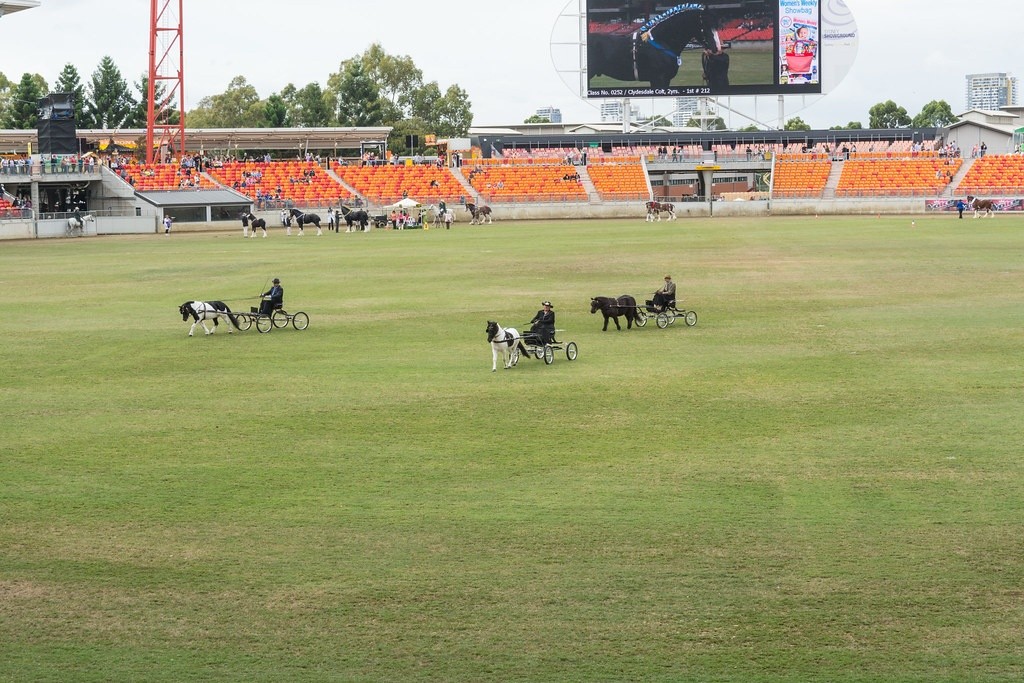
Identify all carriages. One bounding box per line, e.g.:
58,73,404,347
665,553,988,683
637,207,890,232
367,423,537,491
178,298,310,336
486,321,578,372
590,295,698,331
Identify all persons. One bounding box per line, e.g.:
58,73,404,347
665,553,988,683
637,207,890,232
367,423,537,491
530,301,555,344
335,210,342,233
3,154,103,173
460,194,466,205
356,209,371,232
241,213,248,238
105,141,1024,210
438,199,447,213
652,274,677,312
328,207,335,230
73,207,84,229
285,216,292,236
163,214,172,236
785,27,809,41
391,209,427,229
281,209,288,227
956,199,965,218
12,197,20,207
258,279,284,314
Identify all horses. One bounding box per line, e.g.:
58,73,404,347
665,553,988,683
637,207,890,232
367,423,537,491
967,195,995,219
464,202,493,225
588,3,722,87
65,214,95,237
485,320,531,372
340,204,369,233
644,201,677,223
590,295,642,332
178,300,240,337
429,203,456,228
289,208,323,237
246,213,267,239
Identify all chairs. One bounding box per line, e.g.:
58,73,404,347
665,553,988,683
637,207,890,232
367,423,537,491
772,150,1024,197
0,196,20,216
716,18,773,40
501,139,943,156
588,20,645,33
115,156,650,208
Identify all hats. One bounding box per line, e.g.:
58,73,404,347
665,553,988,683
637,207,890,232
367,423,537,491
542,301,553,308
272,278,280,283
664,274,671,279
241,213,247,217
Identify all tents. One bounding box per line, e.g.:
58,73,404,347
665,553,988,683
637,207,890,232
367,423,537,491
393,198,422,225
1013,126,1024,153
